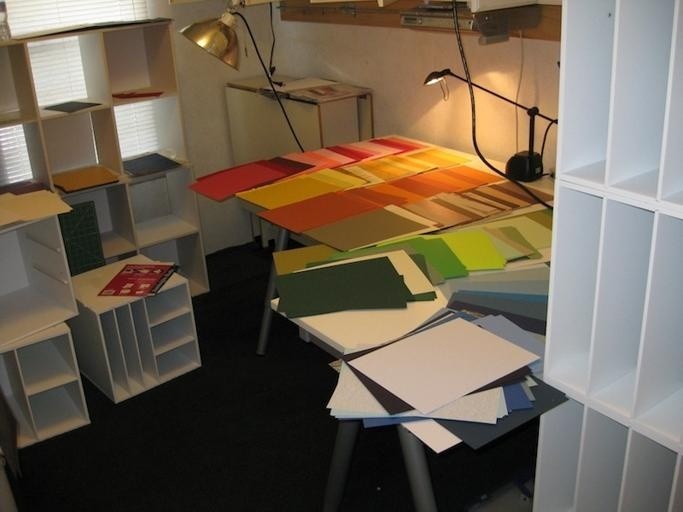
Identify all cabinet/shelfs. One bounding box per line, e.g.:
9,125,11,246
71,254,202,405
0,17,210,299
0,212,81,350
532,1,683,512
0,322,91,449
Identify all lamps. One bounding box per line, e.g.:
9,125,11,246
179,5,241,71
424,66,557,181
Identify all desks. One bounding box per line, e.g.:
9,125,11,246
189,131,554,512
223,73,374,165
